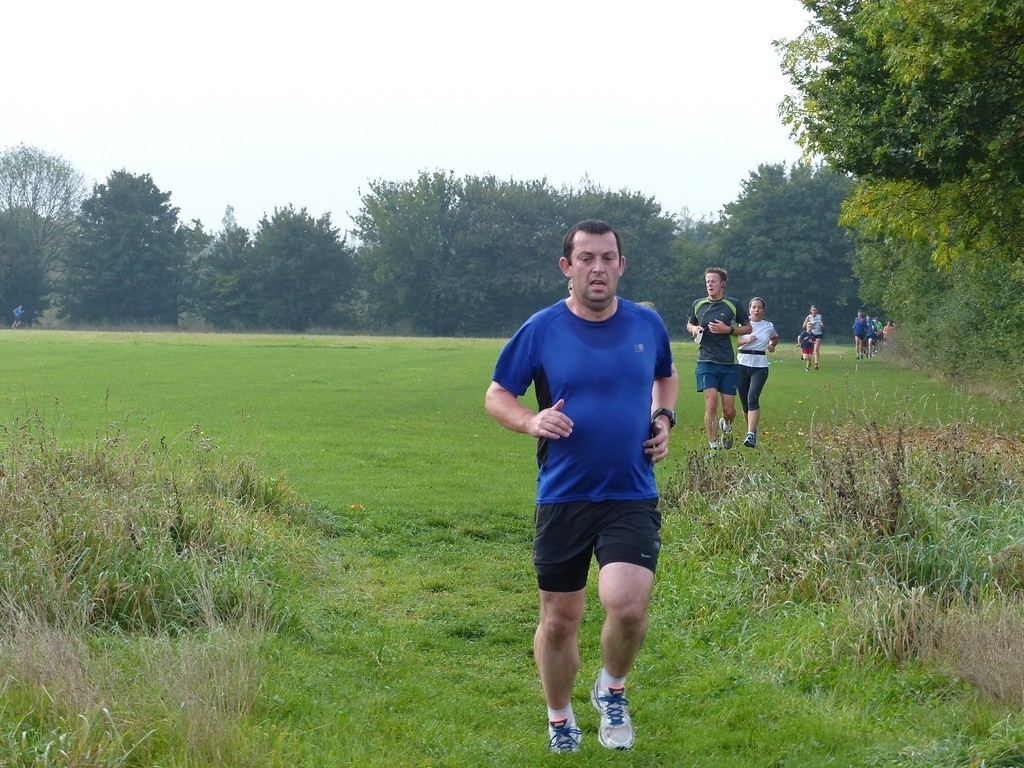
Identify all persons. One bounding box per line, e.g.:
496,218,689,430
685,268,751,454
796,322,817,372
734,295,780,447
484,219,680,757
852,310,898,360
803,305,825,370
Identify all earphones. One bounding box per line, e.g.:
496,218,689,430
722,283,724,287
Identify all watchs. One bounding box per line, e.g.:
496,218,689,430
649,407,677,429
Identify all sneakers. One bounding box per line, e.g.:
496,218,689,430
719,418,733,449
591,668,636,751
547,717,583,756
743,432,756,446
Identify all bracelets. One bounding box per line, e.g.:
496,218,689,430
729,326,734,335
